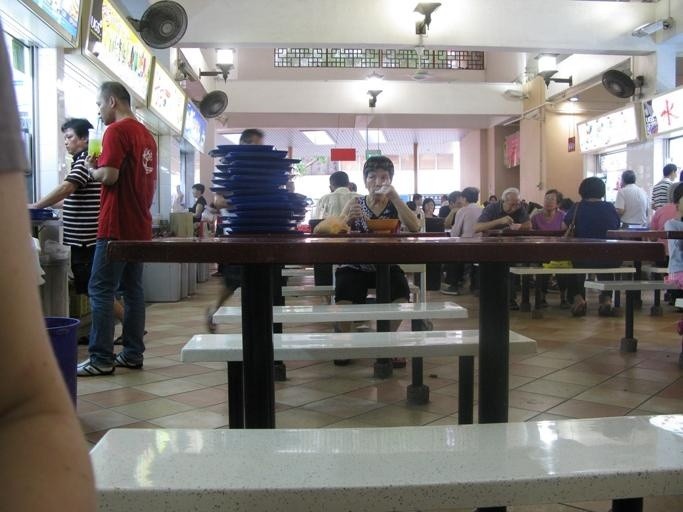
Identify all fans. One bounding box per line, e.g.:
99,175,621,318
193,89,228,118
389,35,458,83
602,69,644,98
127,0,188,50
502,90,531,101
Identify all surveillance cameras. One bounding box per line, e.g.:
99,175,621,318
630,18,665,39
178,60,200,83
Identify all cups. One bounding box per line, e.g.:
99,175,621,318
86,129,103,158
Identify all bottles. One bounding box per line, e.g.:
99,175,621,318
351,197,362,233
211,217,216,233
159,220,168,237
198,212,207,238
415,206,425,233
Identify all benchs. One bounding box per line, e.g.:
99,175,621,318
674,298,682,337
583,280,683,355
90,413,683,512
212,300,469,407
641,263,672,317
281,269,314,277
180,329,540,429
232,281,420,298
508,265,638,315
284,264,314,269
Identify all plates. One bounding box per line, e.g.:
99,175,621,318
626,227,648,230
26,209,55,219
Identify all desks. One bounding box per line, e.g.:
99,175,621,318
107,229,683,512
109,230,682,512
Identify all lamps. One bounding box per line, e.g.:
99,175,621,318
631,17,673,38
533,51,573,90
366,70,384,107
412,0,442,35
199,47,236,84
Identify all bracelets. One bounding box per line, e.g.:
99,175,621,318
87,168,95,182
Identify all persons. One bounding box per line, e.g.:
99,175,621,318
205,129,295,331
0,20,98,512
188,184,223,277
407,170,648,316
333,156,423,367
648,164,683,312
311,171,363,220
77,81,158,377
28,118,147,348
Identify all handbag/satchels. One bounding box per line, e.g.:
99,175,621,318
564,223,576,236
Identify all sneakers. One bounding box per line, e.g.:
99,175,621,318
440,286,459,295
77,358,115,377
114,352,143,369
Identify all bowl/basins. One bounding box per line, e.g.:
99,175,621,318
367,219,398,235
508,223,521,231
